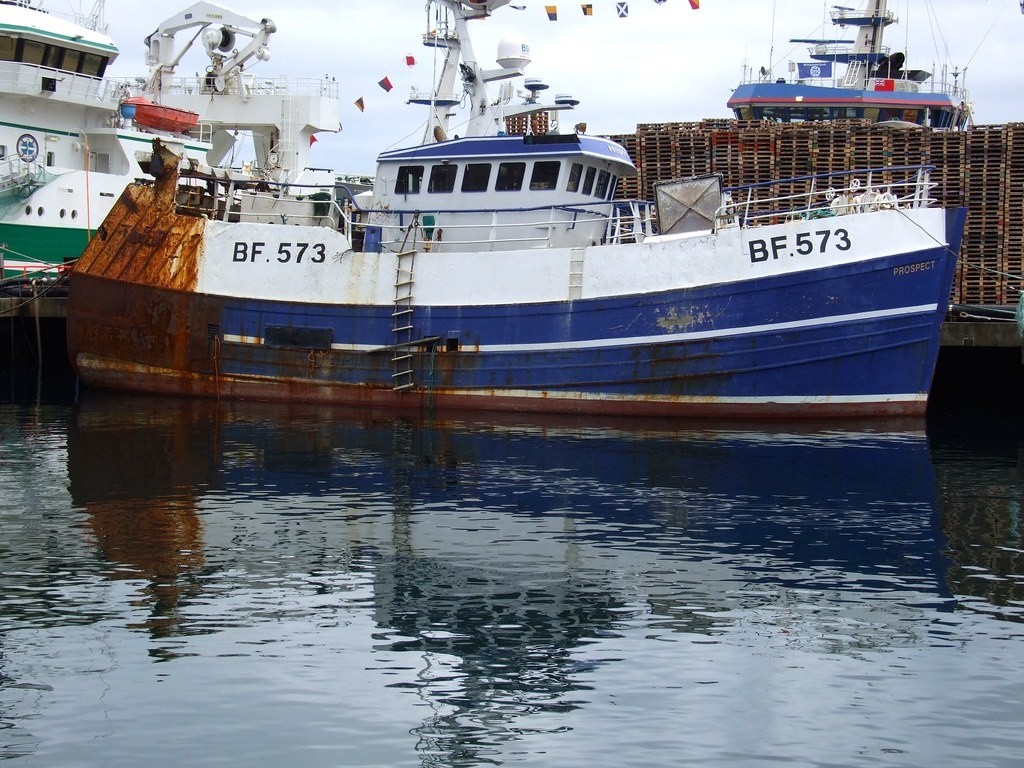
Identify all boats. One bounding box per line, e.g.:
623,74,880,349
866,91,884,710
60,0,971,430
718,0,972,128
1,0,232,286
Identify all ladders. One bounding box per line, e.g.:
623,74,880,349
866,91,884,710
385,207,423,394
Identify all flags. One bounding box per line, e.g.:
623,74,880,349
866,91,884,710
797,62,832,78
509,0,700,22
310,30,437,147
875,80,894,90
